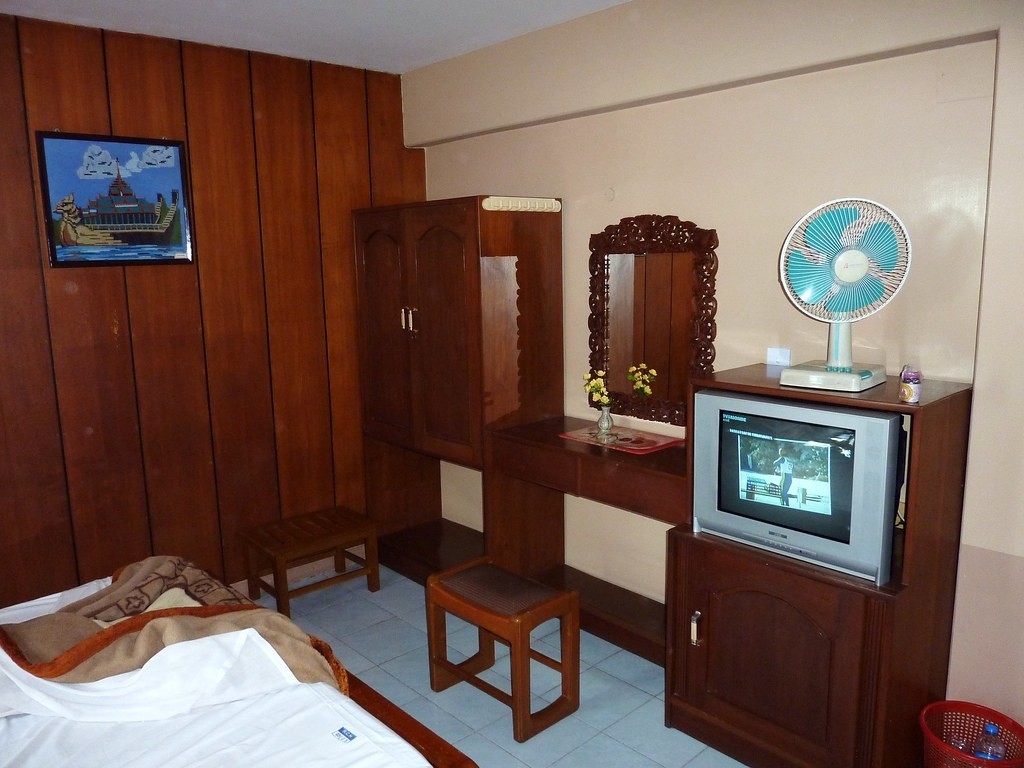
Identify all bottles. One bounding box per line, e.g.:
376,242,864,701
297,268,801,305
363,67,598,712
974,724,1006,762
947,735,972,755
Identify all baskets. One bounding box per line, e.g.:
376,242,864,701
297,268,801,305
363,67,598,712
919,700,1024,768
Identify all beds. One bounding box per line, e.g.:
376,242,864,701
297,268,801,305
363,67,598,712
0,556,479,768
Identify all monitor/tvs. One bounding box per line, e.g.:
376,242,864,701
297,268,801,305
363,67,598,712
693,389,907,588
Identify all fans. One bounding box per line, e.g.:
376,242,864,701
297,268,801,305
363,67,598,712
778,197,913,394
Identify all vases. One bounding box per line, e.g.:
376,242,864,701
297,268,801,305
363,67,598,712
597,407,617,443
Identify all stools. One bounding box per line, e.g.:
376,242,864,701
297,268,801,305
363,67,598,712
426,555,581,743
246,505,381,618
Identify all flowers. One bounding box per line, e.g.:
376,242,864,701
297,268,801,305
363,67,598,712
628,363,658,398
584,370,611,406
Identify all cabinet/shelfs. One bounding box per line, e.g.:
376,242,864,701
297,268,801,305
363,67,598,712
351,195,566,592
492,414,687,528
664,364,972,768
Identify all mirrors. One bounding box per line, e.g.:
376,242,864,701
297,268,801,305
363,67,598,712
589,214,719,426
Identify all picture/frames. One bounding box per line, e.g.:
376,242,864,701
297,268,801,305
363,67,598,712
35,130,194,267
